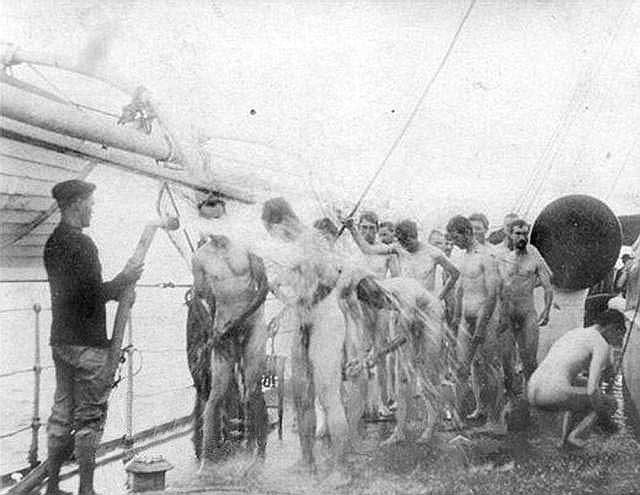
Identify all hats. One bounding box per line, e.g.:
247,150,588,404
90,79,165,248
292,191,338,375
51,180,97,208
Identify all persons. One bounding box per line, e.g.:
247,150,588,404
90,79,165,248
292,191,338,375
44,180,145,495
